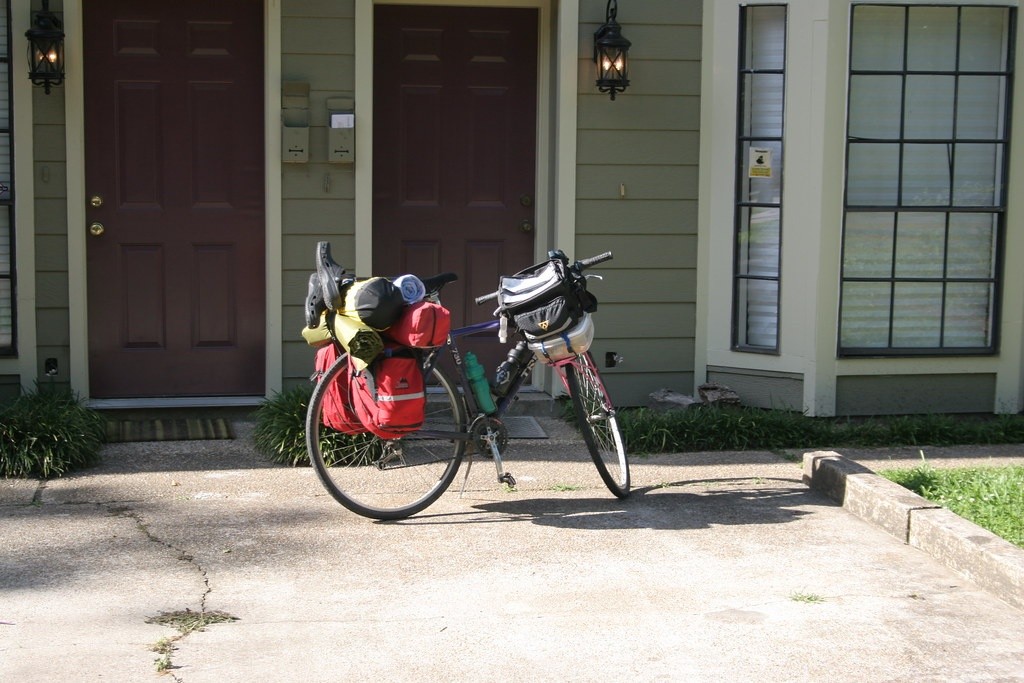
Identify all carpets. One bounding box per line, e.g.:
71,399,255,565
99,417,234,443
404,414,548,439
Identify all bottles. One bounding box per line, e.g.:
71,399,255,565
463,351,497,414
490,341,526,397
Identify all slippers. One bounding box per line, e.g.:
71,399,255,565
316,239,347,310
305,274,327,328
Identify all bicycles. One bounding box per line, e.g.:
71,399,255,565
304,250,631,521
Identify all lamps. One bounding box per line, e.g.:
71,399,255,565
592,0,631,101
24,0,65,95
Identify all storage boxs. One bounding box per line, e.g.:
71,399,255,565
527,311,595,363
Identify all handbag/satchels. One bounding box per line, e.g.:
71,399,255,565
313,345,369,434
347,355,426,440
386,300,451,349
